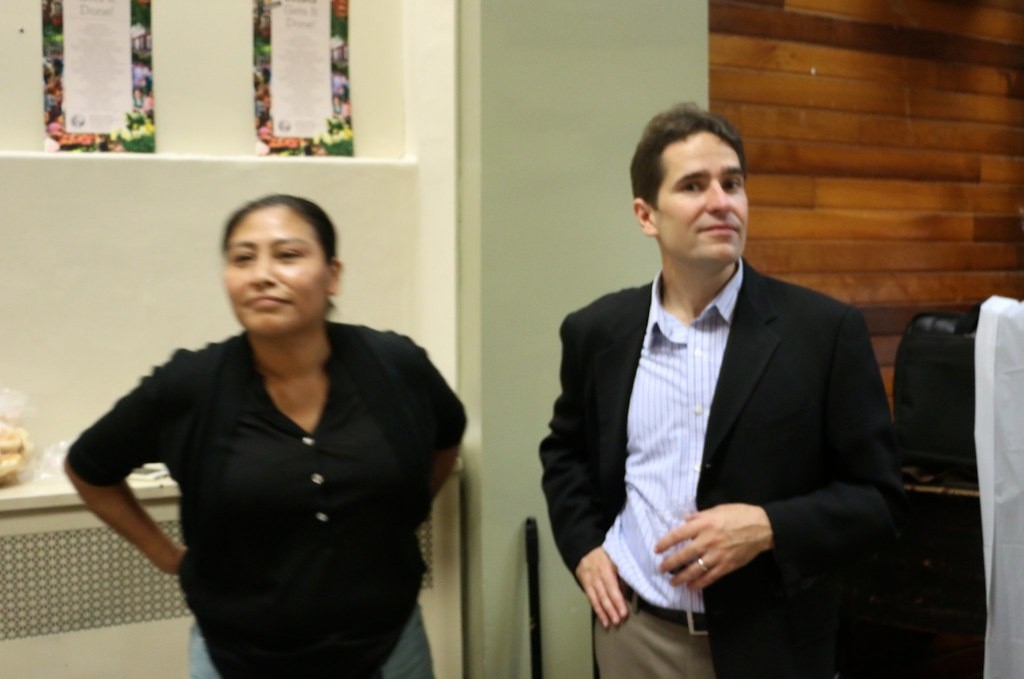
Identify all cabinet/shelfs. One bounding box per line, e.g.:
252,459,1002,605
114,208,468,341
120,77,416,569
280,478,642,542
0,449,464,679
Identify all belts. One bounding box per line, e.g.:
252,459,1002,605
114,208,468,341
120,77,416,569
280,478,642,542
618,578,708,636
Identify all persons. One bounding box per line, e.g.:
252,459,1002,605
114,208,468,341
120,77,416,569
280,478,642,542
64,195,467,679
537,97,909,679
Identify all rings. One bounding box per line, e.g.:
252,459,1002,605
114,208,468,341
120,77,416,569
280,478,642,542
697,557,710,575
